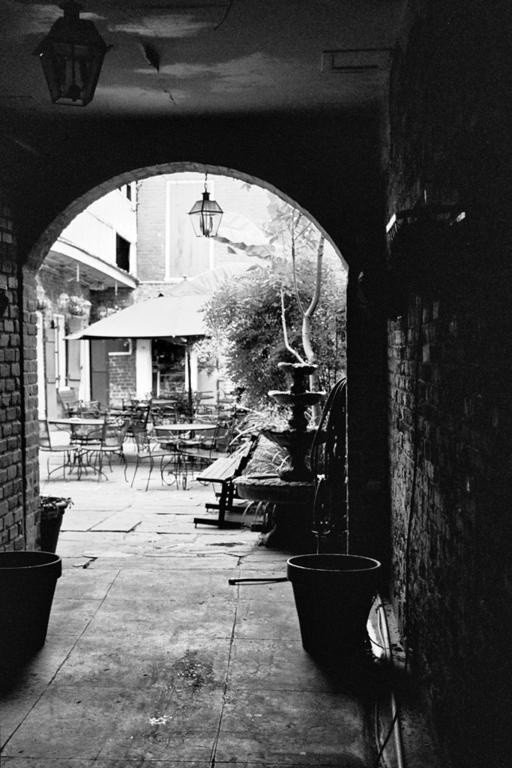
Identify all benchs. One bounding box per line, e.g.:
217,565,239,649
193,428,256,529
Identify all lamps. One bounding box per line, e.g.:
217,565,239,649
40,0,109,106
190,192,223,238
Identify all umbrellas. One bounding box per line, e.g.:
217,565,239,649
59,273,219,441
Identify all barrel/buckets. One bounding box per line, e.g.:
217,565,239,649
1,551,62,654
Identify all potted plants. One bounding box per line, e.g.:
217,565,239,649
41,496,70,559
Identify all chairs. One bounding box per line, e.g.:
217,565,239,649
38,387,238,493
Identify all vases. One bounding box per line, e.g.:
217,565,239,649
0,551,59,678
